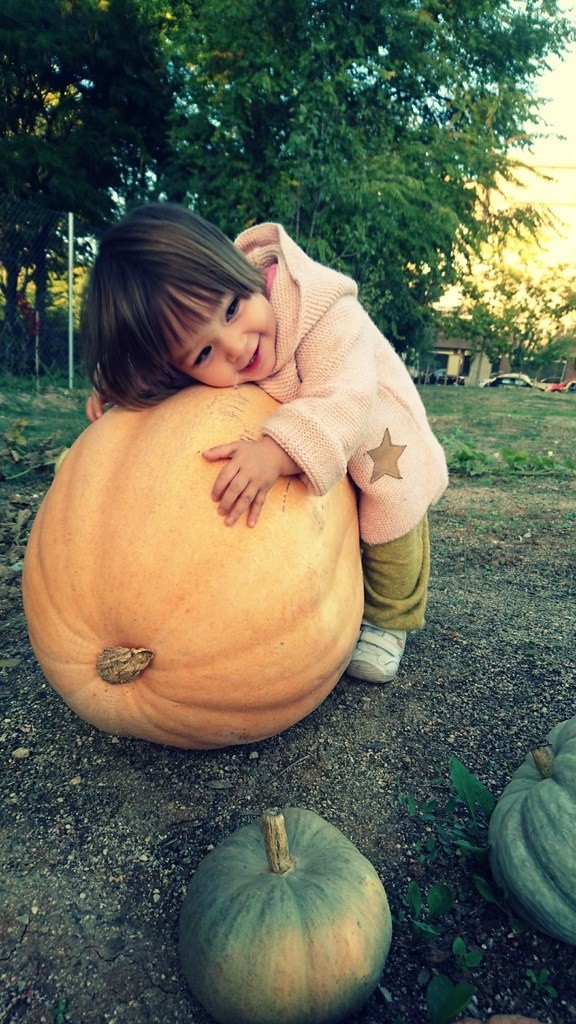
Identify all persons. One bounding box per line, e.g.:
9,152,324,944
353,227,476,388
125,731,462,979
87,203,449,686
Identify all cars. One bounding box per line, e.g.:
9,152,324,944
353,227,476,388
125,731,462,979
549,378,576,393
482,373,546,392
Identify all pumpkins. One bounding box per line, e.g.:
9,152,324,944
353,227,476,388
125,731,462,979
487,718,576,945
21,383,365,747
180,807,392,1024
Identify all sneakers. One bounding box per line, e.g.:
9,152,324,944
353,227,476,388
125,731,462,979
345,616,406,682
